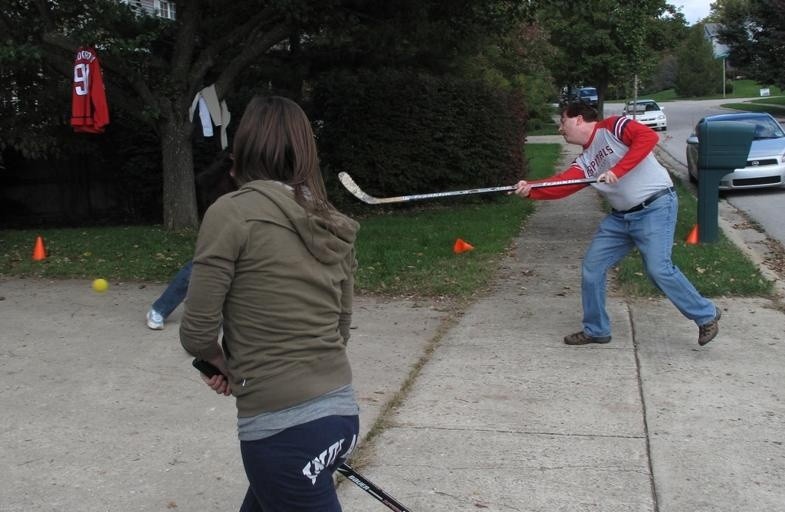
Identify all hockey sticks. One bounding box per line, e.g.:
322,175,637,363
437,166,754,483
338,172,608,206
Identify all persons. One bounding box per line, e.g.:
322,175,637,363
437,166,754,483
509,100,720,347
144,262,191,332
178,95,362,510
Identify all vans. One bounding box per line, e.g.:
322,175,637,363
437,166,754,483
578,87,598,106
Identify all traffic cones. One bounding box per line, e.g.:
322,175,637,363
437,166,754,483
454,237,474,252
685,225,699,243
32,237,46,262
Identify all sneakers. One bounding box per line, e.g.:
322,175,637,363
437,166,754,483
146,310,164,330
564,331,611,345
698,307,721,346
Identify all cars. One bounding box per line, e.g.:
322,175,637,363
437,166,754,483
624,99,667,131
686,111,785,195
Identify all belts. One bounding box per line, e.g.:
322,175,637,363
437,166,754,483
611,186,676,214
